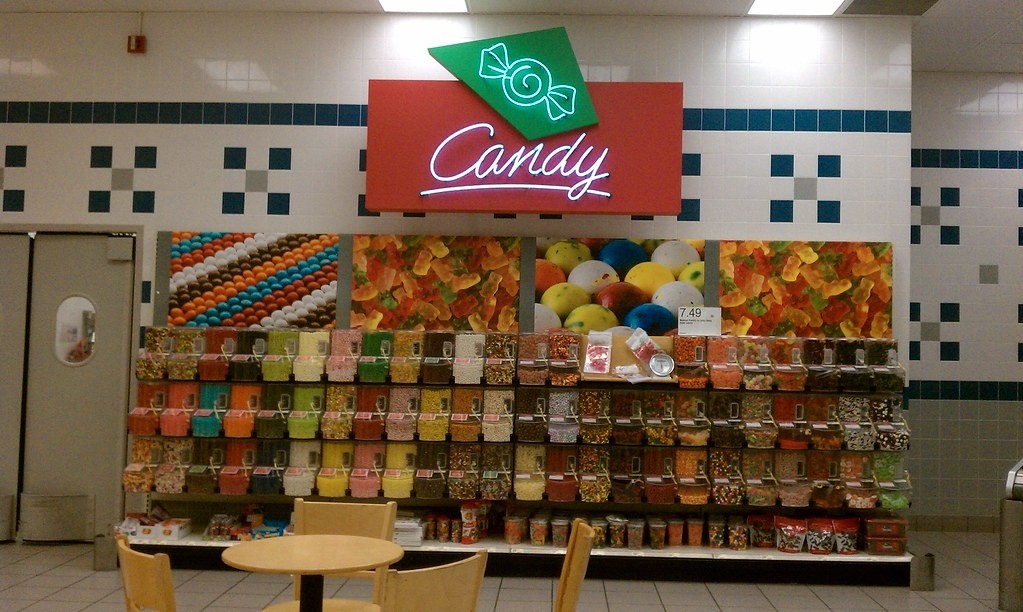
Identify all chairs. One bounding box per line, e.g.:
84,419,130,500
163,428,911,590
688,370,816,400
554,518,595,612
382,548,488,612
114,534,177,612
260,498,397,612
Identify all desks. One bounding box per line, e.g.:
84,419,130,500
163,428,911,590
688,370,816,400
222,534,404,612
